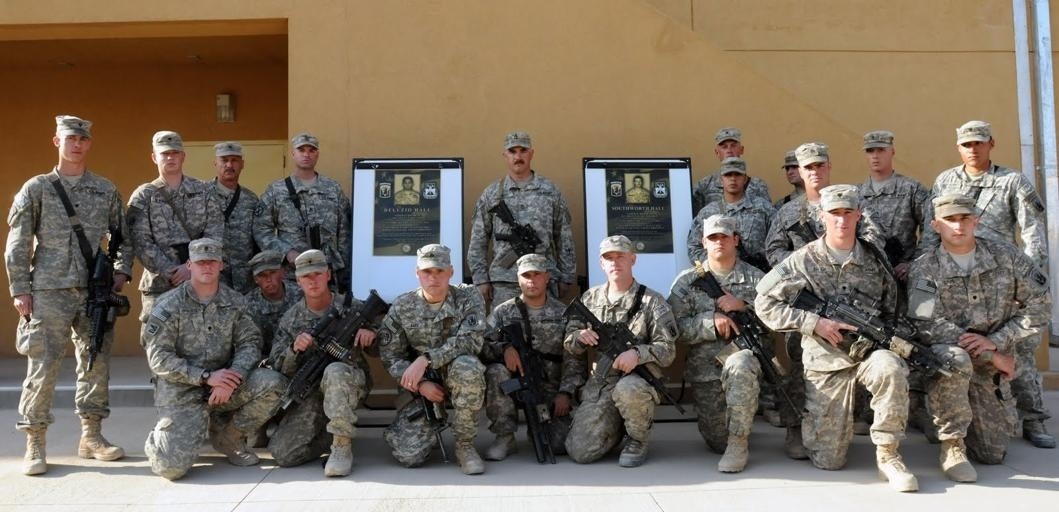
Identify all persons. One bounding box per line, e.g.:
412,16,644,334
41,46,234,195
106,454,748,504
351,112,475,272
267,249,375,477
666,214,766,473
563,235,680,467
141,238,290,480
922,121,1057,448
626,173,650,202
855,130,930,313
126,130,226,337
907,194,1054,483
395,174,420,205
693,127,774,221
771,150,806,211
204,142,262,294
377,243,488,476
466,130,578,316
482,253,569,462
2,114,136,477
687,157,777,274
252,132,352,288
766,141,888,460
754,184,919,493
243,248,304,366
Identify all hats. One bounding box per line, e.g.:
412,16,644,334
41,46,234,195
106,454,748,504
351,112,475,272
720,156,746,175
703,214,736,238
416,244,451,270
780,150,798,169
294,249,328,277
152,130,184,153
516,253,547,276
248,250,284,277
862,131,895,149
502,131,531,150
931,194,976,218
188,237,223,264
214,142,243,157
818,183,860,211
291,134,319,149
56,115,94,139
956,120,992,145
713,128,741,144
795,142,829,166
599,235,632,256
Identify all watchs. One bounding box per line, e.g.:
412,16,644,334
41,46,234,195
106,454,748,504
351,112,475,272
200,369,212,387
979,349,996,365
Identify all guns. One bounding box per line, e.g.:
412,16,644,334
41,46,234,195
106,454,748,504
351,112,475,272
561,298,687,418
688,271,803,422
790,286,952,381
487,200,544,270
497,322,557,466
85,226,128,372
404,345,452,467
273,288,392,425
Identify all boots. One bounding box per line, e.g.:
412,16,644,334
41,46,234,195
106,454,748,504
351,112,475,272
852,411,869,434
324,435,353,477
454,441,484,475
907,390,931,432
21,428,48,475
618,437,649,467
717,432,749,472
939,439,977,482
76,418,125,461
763,408,784,426
485,432,518,461
784,427,807,459
1022,417,1055,448
212,420,260,466
875,444,920,492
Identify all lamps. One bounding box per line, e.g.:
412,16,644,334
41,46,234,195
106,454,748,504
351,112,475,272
216,94,237,121
